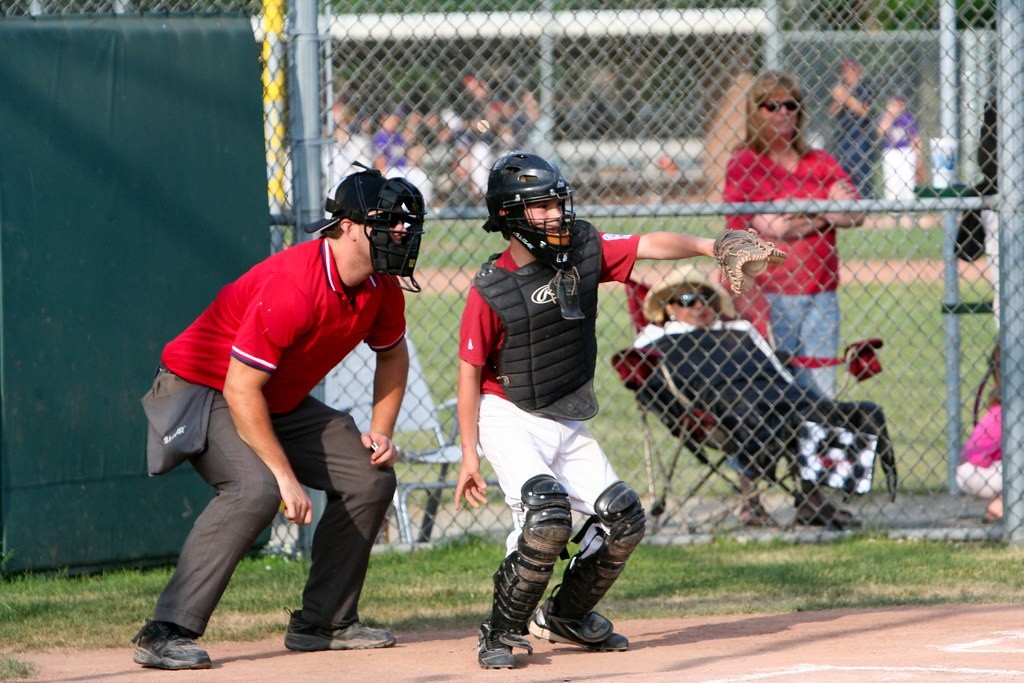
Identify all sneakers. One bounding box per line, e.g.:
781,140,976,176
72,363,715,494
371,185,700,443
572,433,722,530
284,611,396,652
477,622,532,670
531,599,629,652
134,633,214,670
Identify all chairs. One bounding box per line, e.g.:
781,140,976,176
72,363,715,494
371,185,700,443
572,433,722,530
309,333,500,546
610,347,842,532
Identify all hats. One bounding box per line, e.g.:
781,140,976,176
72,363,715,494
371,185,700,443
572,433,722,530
304,171,403,233
643,263,732,323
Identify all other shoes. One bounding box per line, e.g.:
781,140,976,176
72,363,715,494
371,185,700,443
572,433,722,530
732,496,779,528
793,492,861,527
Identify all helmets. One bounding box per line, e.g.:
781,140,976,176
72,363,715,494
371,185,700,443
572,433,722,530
484,154,578,272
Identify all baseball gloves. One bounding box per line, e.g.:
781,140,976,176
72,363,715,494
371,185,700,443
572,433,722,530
713,227,786,298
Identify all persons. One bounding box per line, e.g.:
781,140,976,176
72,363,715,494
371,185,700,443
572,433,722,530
635,262,884,528
329,67,539,210
132,171,410,668
828,63,916,199
722,72,864,528
955,344,1004,525
453,156,785,670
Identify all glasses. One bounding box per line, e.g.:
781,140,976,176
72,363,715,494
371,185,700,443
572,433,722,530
759,99,798,113
668,291,712,308
